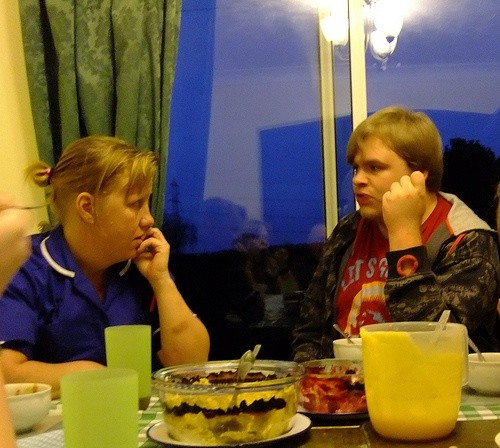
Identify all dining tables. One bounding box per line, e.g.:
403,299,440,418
10,382,500,448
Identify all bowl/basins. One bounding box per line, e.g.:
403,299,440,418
466,352,500,396
333,338,363,359
298,359,361,384
4,383,52,435
153,359,309,446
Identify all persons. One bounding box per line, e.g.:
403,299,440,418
192,195,328,324
297,107,499,365
0,137,210,410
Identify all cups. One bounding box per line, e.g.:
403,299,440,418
359,321,469,442
60,367,140,448
104,325,152,411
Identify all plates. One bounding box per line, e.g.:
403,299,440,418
146,413,312,448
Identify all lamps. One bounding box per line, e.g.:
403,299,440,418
322,0,404,63
